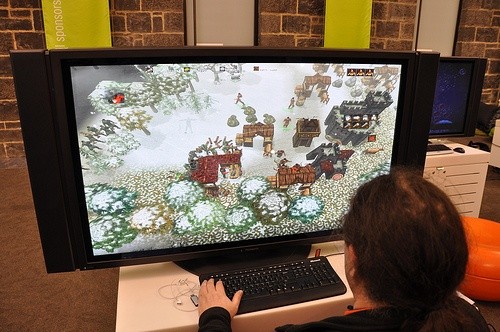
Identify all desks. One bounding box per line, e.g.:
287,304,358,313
115,238,355,332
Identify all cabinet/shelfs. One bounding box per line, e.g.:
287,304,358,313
423,139,491,217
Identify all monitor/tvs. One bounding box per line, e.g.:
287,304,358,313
427,56,487,144
9,45,440,275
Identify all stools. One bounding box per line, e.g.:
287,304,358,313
457,218,500,301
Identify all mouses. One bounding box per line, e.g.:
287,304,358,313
453,147,465,153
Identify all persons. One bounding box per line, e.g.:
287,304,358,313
196,166,491,332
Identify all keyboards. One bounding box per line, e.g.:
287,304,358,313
199,256,347,315
425,144,454,156
